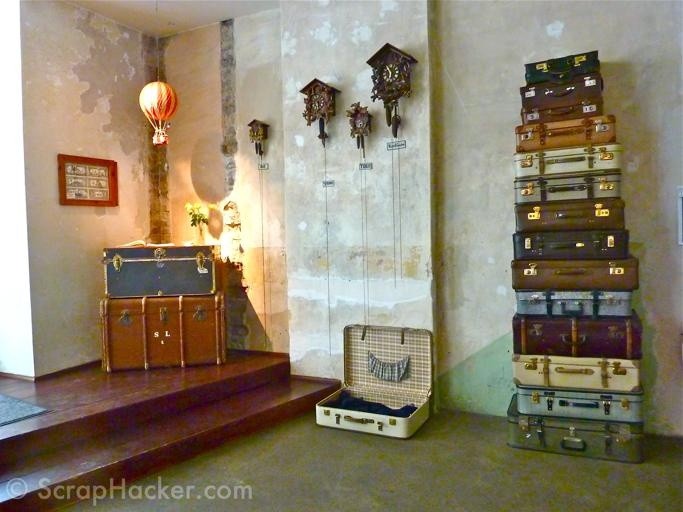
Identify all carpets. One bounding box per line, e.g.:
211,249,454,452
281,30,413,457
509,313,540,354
0,394,48,428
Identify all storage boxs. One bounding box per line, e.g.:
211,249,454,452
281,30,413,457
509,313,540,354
100,246,225,374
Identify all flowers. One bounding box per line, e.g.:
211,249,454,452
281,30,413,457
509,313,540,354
185,203,211,237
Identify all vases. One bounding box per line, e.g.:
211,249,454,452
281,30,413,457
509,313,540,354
196,224,206,245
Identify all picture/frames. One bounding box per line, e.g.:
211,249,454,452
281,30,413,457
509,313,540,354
57,154,119,207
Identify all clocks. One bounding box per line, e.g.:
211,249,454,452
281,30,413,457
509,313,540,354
248,119,270,159
298,42,419,159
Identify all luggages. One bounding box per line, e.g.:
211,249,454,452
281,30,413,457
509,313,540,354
315,323,436,438
